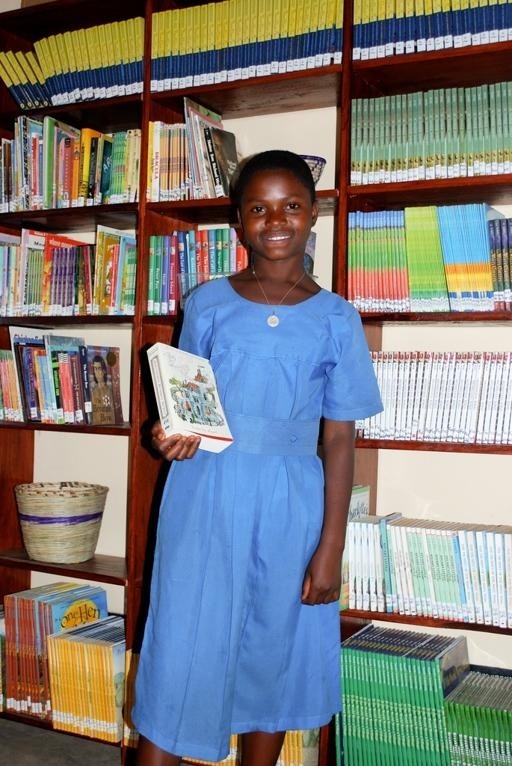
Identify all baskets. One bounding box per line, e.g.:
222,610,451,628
14,481,109,563
296,154,326,185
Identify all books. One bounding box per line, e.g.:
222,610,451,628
0,325,122,425
343,485,512,632
147,226,315,321
353,350,512,446
180,713,319,766
144,338,233,456
1,112,141,211
334,626,511,766
147,93,243,202
347,203,512,319
350,0,511,64
154,0,344,93
0,223,136,317
0,580,141,748
350,79,512,185
1,12,142,104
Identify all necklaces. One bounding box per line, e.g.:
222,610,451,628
251,266,306,327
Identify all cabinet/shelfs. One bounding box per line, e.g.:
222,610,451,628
2,1,512,740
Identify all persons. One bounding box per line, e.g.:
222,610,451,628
128,152,383,765
